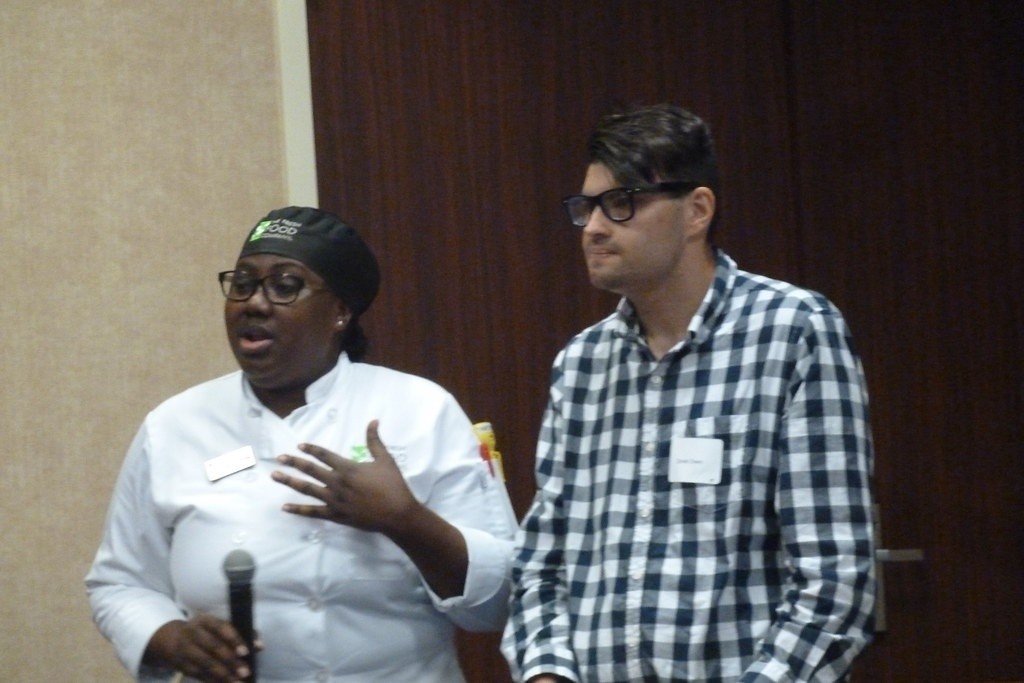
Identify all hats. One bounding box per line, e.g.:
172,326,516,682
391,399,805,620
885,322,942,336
237,205,382,314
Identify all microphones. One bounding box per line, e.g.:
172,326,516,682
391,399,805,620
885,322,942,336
223,550,258,683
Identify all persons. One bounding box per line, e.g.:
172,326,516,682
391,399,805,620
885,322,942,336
500,104,891,681
81,205,523,683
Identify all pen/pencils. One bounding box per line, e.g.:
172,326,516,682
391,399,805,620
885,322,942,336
478,442,496,478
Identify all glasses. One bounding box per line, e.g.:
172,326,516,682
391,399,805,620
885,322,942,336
561,180,693,229
219,271,340,305
